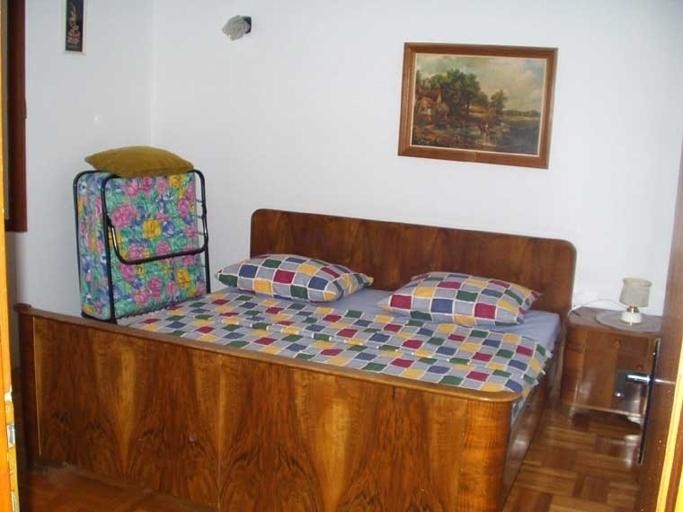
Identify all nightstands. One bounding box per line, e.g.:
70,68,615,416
560,305,663,430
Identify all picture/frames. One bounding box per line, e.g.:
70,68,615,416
398,42,557,169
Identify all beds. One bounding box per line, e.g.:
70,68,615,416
12,209,577,512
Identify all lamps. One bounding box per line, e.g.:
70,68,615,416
619,278,651,324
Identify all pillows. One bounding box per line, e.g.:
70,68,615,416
214,253,375,303
84,146,193,177
377,271,543,326
